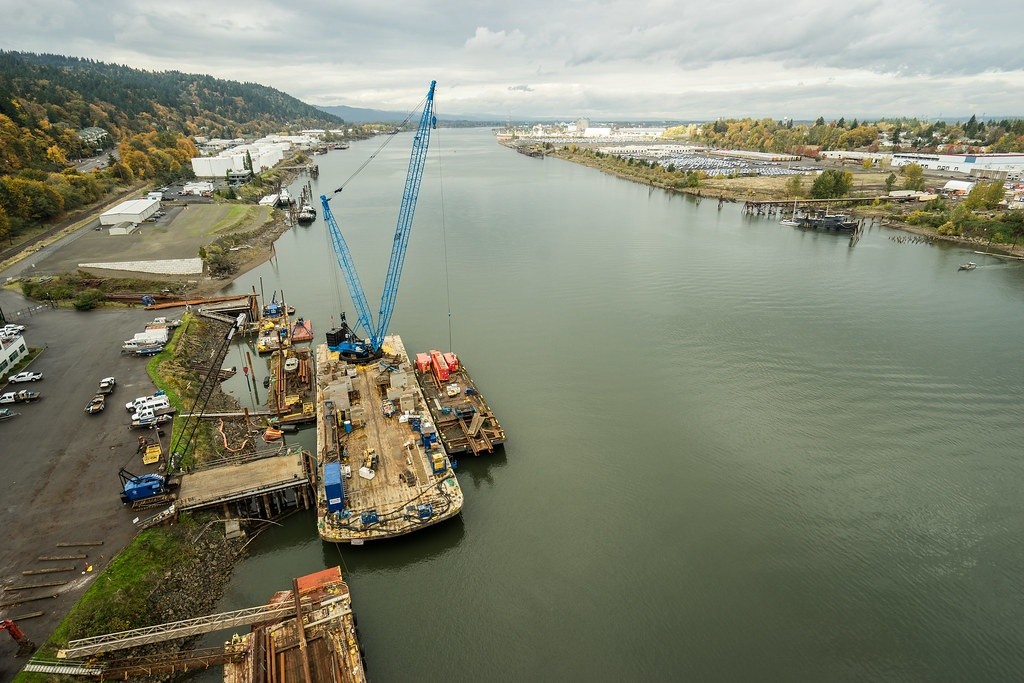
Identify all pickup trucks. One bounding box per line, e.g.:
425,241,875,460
8,370,43,385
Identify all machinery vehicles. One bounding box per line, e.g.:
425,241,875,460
322,80,455,367
118,313,260,509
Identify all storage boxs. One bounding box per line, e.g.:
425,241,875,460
359,467,375,480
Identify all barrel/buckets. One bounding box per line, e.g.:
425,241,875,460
344,420,351,432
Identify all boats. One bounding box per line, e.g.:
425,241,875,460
313,334,466,546
254,275,319,428
411,347,507,456
120,316,185,356
83,394,106,414
0,408,22,420
958,261,978,269
96,376,115,395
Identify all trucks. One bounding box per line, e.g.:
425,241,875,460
123,393,173,426
0,390,40,405
